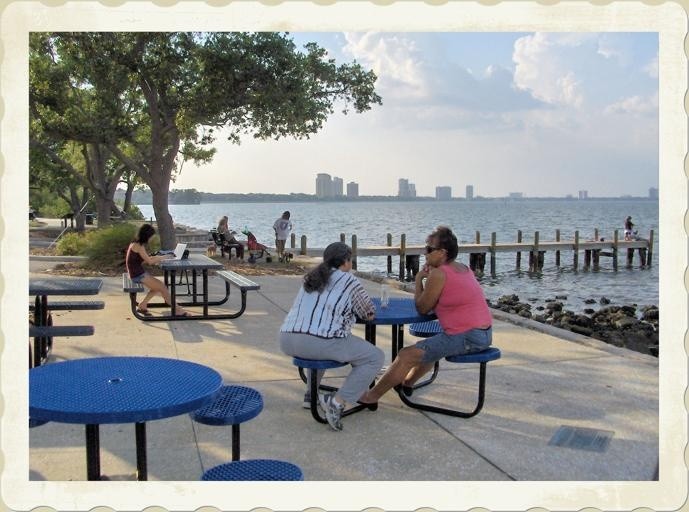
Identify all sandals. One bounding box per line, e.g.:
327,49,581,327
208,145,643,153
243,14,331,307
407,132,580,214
355,399,379,412
393,382,412,398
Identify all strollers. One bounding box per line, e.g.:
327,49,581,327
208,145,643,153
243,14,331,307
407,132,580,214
241,226,273,263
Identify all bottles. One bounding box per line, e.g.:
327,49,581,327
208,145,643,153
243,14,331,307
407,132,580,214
379,282,390,308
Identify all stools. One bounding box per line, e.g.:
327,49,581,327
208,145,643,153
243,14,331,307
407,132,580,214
192,384,264,458
399,347,499,420
295,357,365,421
405,323,442,389
204,459,301,480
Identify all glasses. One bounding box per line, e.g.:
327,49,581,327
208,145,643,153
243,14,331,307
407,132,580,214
425,246,440,253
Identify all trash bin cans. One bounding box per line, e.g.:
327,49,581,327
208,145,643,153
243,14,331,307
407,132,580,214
86,214,93,224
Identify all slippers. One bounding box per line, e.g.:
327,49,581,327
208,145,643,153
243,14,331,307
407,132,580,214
136,308,152,316
175,312,192,317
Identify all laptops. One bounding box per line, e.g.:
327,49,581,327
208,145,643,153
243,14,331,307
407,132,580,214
160,243,187,261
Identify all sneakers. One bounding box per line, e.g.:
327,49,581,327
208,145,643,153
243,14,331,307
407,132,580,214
319,392,345,432
303,393,322,410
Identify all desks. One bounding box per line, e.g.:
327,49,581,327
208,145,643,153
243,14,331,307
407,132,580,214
29,355,222,480
150,252,214,317
353,297,433,409
28,280,102,362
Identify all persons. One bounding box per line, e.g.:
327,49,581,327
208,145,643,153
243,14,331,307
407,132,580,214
29,206,36,220
597,238,604,253
217,219,241,257
273,211,291,262
126,224,191,317
623,215,640,242
223,216,229,231
357,227,492,411
279,241,376,433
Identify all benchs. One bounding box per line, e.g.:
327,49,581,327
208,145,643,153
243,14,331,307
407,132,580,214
27,302,103,319
118,270,146,322
208,231,244,258
31,324,94,362
212,268,256,315
